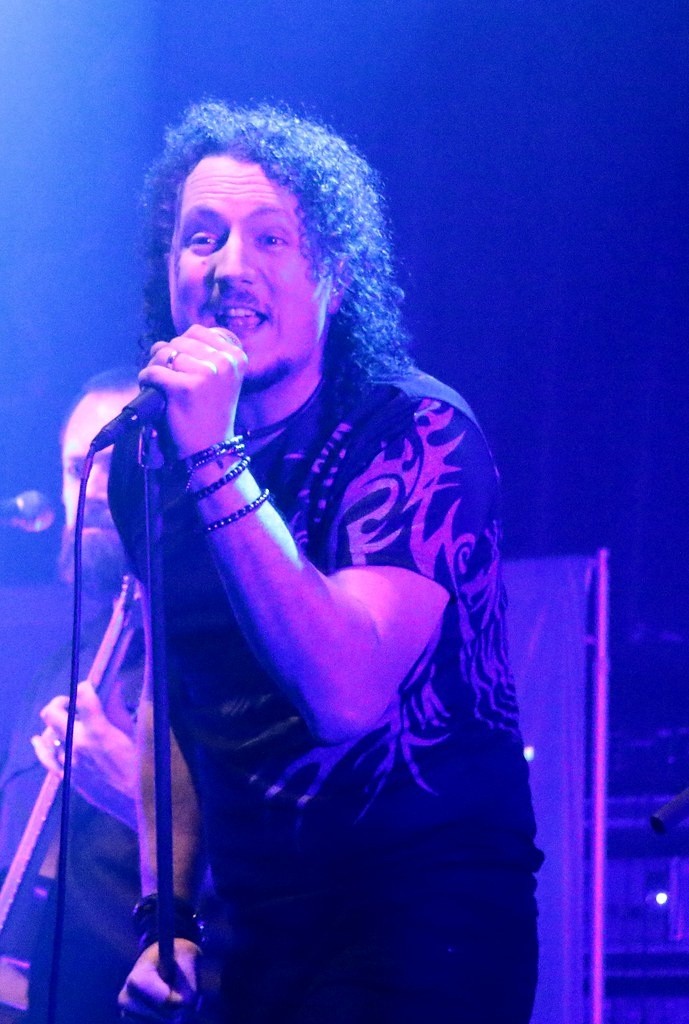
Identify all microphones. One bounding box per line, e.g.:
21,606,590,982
85,323,241,453
0,487,55,532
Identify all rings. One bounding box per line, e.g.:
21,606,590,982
54,739,67,769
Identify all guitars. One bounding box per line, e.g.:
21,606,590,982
0,573,147,1023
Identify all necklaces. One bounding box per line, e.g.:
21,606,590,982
239,380,320,442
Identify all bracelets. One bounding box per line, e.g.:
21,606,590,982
178,433,246,474
205,487,272,538
134,893,203,944
188,455,251,499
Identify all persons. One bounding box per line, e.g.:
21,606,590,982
0,367,145,1024
104,92,546,1024
